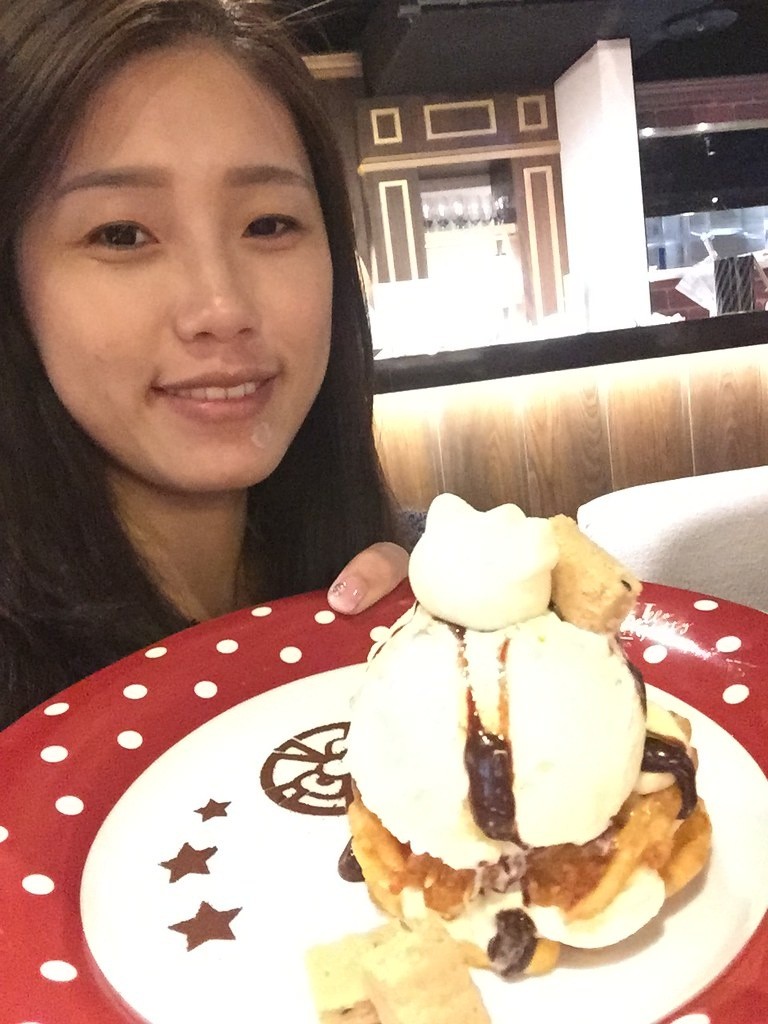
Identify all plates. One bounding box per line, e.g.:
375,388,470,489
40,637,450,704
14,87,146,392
0,574,768,1024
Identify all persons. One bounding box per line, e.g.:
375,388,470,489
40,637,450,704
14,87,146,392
0,0,426,732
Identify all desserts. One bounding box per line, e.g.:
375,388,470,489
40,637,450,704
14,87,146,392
347,489,710,975
